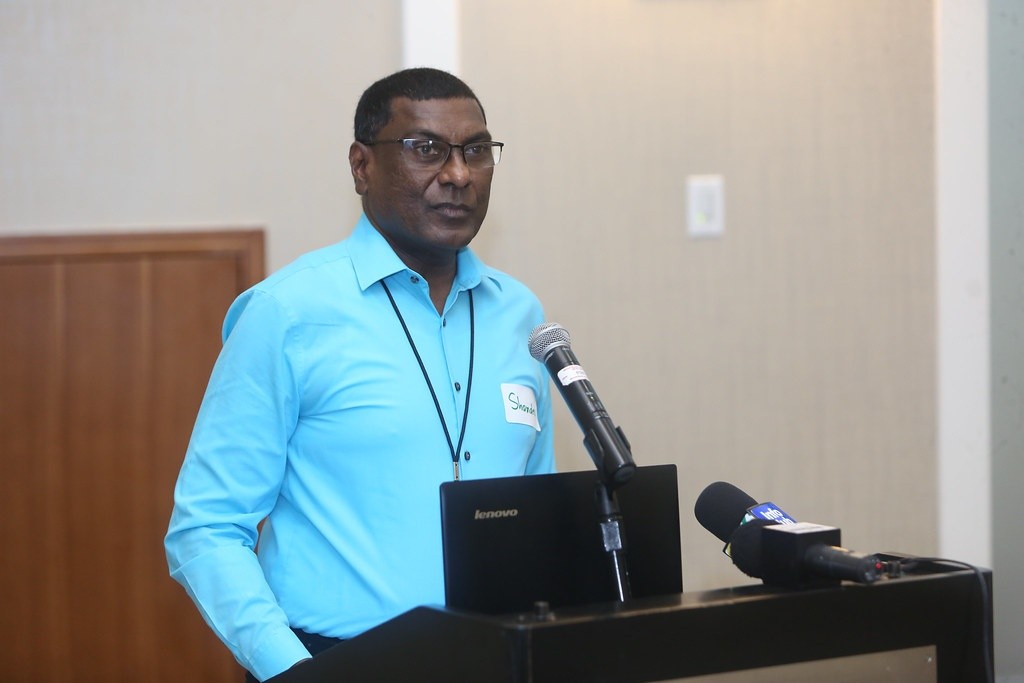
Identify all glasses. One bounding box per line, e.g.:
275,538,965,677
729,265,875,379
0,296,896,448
360,137,504,171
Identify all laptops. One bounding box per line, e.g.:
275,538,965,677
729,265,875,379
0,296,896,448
439,464,683,614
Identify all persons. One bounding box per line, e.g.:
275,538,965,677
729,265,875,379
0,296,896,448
165,68,558,683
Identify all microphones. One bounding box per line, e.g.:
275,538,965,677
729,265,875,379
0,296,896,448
529,322,636,485
694,481,881,585
